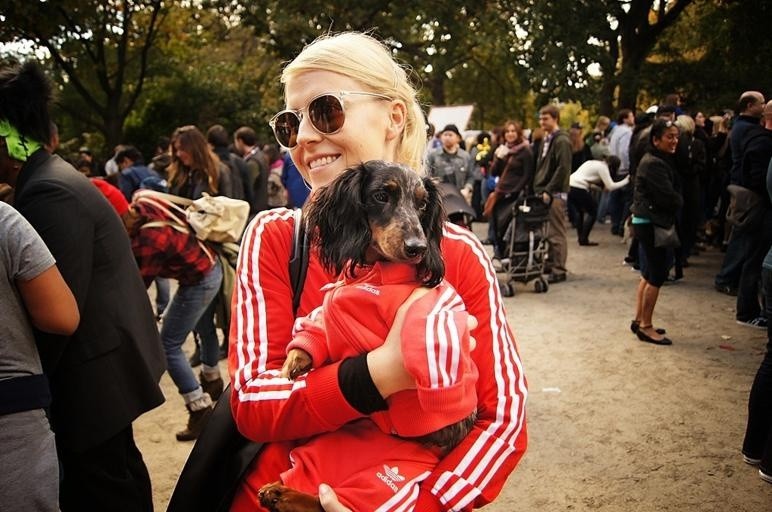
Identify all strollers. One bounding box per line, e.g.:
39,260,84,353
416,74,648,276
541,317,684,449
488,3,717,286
436,182,477,233
491,189,551,296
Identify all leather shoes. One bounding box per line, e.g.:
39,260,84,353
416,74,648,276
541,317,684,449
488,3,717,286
630,320,672,346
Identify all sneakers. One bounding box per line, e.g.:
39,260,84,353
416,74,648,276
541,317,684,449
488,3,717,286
715,281,737,295
189,342,227,366
736,315,768,330
547,273,566,284
580,240,600,247
481,239,494,245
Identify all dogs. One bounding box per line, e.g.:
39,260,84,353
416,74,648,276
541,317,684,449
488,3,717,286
621,214,635,257
258,160,477,512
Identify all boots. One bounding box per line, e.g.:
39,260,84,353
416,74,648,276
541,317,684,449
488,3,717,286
175,393,213,442
199,370,224,402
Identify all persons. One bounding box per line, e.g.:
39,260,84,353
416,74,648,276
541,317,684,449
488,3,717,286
427,106,629,281
228,30,527,511
590,91,772,481
1,53,312,512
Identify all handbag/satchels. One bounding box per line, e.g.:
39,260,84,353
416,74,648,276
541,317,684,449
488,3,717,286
163,380,272,510
483,192,497,217
653,225,680,250
184,196,251,245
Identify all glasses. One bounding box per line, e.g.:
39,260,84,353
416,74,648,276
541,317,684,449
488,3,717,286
268,88,394,153
